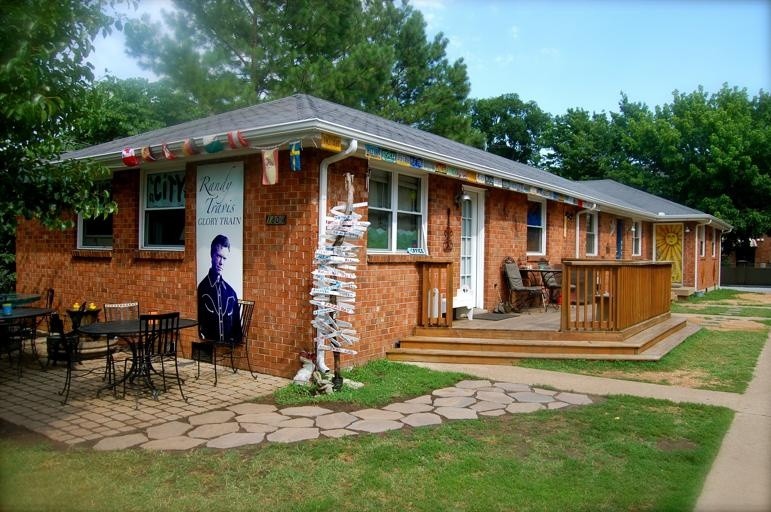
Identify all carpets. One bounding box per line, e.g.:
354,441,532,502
464,313,521,321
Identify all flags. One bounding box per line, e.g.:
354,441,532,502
261,141,302,186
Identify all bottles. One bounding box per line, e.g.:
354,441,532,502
2,304,13,316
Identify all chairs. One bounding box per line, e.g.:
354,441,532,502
118,312,188,409
539,262,562,312
192,301,257,387
503,255,541,314
0,288,200,405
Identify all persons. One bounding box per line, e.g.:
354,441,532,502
197,234,243,345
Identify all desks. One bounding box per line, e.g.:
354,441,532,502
519,269,562,312
